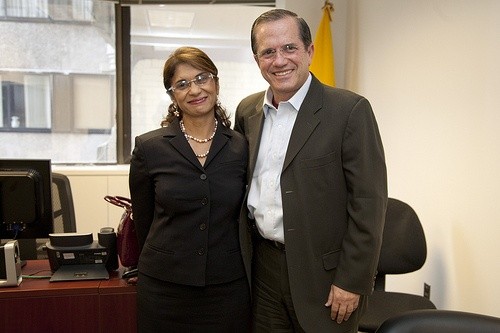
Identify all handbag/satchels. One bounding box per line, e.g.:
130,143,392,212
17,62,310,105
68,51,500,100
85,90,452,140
104,196,140,267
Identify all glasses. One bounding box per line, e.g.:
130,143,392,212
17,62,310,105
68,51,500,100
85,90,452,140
168,72,216,94
254,43,310,60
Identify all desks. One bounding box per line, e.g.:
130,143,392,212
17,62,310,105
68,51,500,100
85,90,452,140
0,259,136,333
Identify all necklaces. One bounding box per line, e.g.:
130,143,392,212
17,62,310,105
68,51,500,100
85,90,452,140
179,118,218,157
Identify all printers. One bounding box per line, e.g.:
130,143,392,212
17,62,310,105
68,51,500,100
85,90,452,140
45,226,119,283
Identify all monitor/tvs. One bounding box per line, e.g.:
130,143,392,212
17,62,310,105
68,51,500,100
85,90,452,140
0,158,55,268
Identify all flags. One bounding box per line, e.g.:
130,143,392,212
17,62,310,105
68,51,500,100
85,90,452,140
308,0,336,88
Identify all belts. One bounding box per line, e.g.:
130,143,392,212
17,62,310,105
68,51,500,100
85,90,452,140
267,239,285,251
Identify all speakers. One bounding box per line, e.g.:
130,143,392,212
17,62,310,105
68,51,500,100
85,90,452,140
0,240,23,287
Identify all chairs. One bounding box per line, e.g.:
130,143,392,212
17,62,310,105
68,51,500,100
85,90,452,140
357,199,436,333
38,174,76,259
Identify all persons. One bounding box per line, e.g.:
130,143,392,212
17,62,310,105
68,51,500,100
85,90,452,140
234,7,388,333
129,47,250,333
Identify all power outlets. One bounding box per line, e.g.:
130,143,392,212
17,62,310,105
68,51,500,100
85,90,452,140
423,283,431,301
